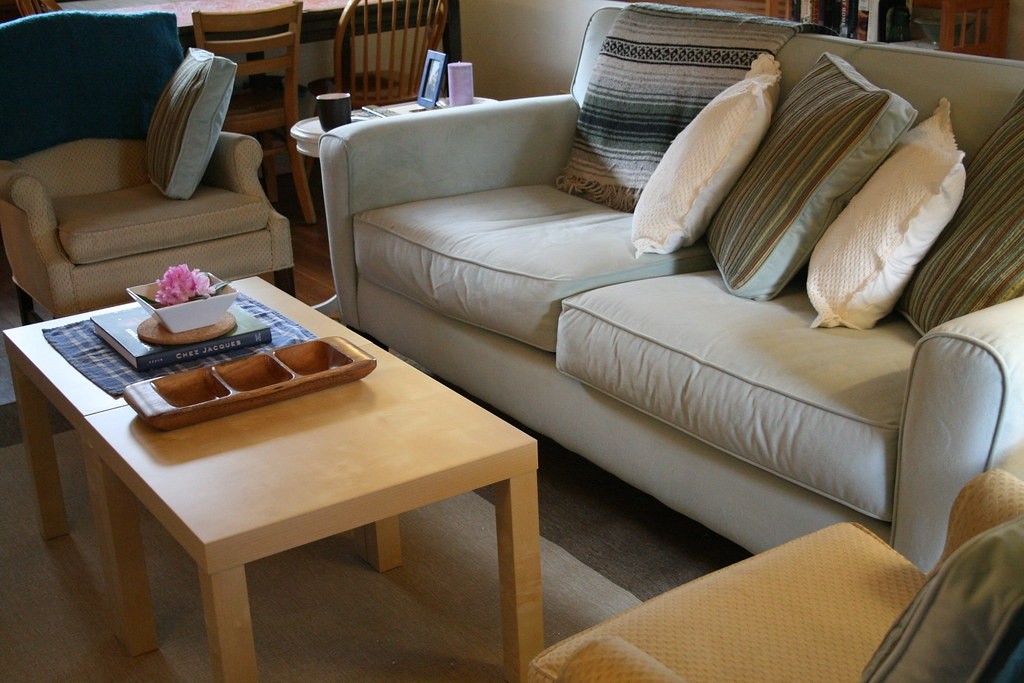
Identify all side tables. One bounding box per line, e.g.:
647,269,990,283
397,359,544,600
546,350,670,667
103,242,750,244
288,96,499,157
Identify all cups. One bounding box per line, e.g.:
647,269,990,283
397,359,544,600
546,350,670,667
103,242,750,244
316,93,352,132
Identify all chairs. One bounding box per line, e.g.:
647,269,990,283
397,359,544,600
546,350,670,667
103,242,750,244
191,0,317,223
528,470,1024,683
307,0,448,181
0,10,294,325
938,0,1010,59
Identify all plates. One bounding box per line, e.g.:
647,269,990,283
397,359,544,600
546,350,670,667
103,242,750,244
124,337,377,433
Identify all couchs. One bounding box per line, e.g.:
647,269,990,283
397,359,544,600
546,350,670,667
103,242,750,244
319,5,1024,575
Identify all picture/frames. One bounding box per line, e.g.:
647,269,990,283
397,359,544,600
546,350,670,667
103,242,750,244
417,50,446,108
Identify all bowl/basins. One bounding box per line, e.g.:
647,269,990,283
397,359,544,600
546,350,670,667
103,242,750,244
126,273,239,334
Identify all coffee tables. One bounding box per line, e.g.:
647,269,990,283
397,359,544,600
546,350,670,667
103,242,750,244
2,275,544,683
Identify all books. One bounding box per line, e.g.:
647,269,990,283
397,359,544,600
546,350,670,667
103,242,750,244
90,300,272,370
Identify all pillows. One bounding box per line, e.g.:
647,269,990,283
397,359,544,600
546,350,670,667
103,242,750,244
898,87,1024,334
707,51,918,302
145,48,238,201
862,513,1024,683
632,53,782,262
807,98,967,331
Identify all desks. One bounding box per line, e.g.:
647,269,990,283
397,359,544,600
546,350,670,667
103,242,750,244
93,0,462,62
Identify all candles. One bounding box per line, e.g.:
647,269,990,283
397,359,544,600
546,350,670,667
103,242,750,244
448,61,474,107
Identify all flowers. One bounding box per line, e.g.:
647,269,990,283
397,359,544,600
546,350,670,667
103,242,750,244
155,263,215,306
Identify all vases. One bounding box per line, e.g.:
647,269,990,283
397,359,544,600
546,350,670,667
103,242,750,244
127,271,239,334
